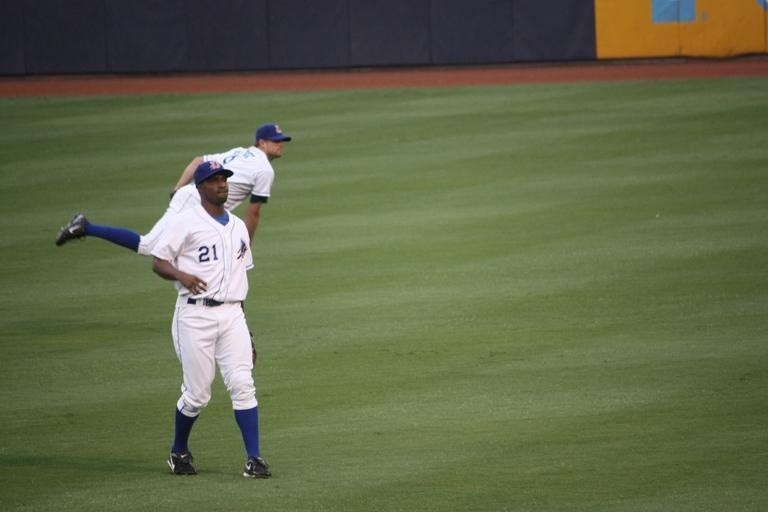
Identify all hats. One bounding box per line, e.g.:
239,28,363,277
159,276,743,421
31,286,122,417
256,124,291,142
194,160,234,186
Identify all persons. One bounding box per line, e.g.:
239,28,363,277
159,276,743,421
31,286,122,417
150,161,271,479
54,124,291,364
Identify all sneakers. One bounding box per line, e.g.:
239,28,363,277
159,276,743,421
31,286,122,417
55,213,87,246
168,450,196,475
243,457,271,478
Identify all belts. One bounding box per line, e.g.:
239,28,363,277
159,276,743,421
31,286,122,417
188,299,223,306
